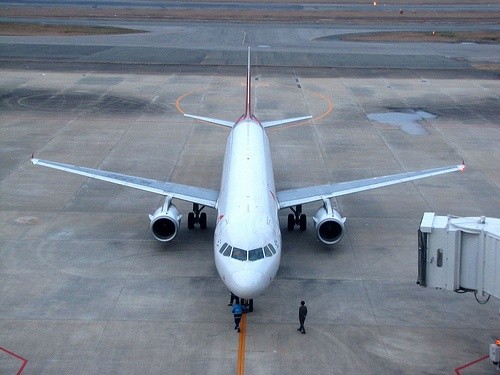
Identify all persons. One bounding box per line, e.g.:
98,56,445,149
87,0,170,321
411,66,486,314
228,292,240,307
297,300,308,334
231,300,247,333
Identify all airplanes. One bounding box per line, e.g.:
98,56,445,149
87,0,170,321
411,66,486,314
30,46,466,313
